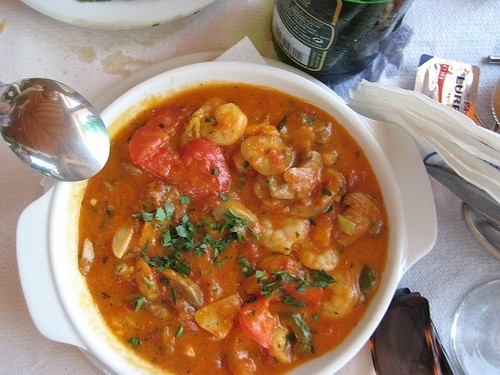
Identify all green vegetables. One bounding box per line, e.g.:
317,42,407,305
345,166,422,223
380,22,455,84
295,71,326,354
127,193,336,349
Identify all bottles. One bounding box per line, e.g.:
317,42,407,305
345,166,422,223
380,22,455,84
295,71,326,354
270,0,414,85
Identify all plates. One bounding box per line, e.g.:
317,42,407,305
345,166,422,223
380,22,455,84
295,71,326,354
450,279,500,375
461,199,500,262
19,0,216,31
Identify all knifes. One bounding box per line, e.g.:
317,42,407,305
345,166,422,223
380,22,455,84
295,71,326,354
425,164,500,229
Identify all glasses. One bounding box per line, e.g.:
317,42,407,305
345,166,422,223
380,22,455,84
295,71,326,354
370,288,456,375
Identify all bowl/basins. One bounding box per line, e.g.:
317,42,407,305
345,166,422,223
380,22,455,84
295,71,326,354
10,62,437,374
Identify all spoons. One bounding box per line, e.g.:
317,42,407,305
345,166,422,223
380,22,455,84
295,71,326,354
0,78,111,179
